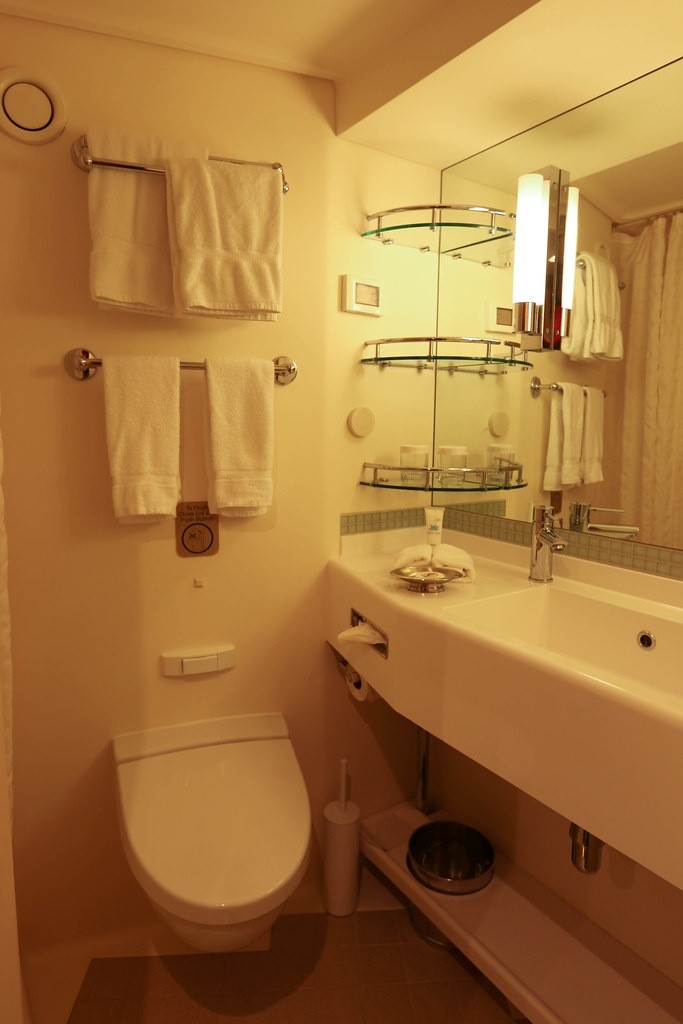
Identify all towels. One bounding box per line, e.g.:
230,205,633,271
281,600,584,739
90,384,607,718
204,354,274,516
562,252,624,362
393,543,476,582
101,354,184,521
84,124,209,313
164,154,285,322
578,386,605,483
543,382,582,491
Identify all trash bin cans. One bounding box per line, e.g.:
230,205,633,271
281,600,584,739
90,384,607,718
408,817,495,953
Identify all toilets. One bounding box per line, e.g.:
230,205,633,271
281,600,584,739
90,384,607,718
110,710,313,952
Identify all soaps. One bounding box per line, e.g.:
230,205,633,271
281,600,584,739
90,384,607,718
416,571,445,581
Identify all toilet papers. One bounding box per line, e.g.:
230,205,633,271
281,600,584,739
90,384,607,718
342,664,381,703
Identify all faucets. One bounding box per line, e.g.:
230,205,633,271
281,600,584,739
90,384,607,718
528,504,569,583
567,502,639,541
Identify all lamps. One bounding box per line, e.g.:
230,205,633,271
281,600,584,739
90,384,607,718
508,162,558,354
550,168,580,351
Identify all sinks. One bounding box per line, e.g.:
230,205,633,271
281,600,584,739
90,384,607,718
443,585,683,890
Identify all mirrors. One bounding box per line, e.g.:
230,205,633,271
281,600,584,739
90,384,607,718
428,57,683,552
